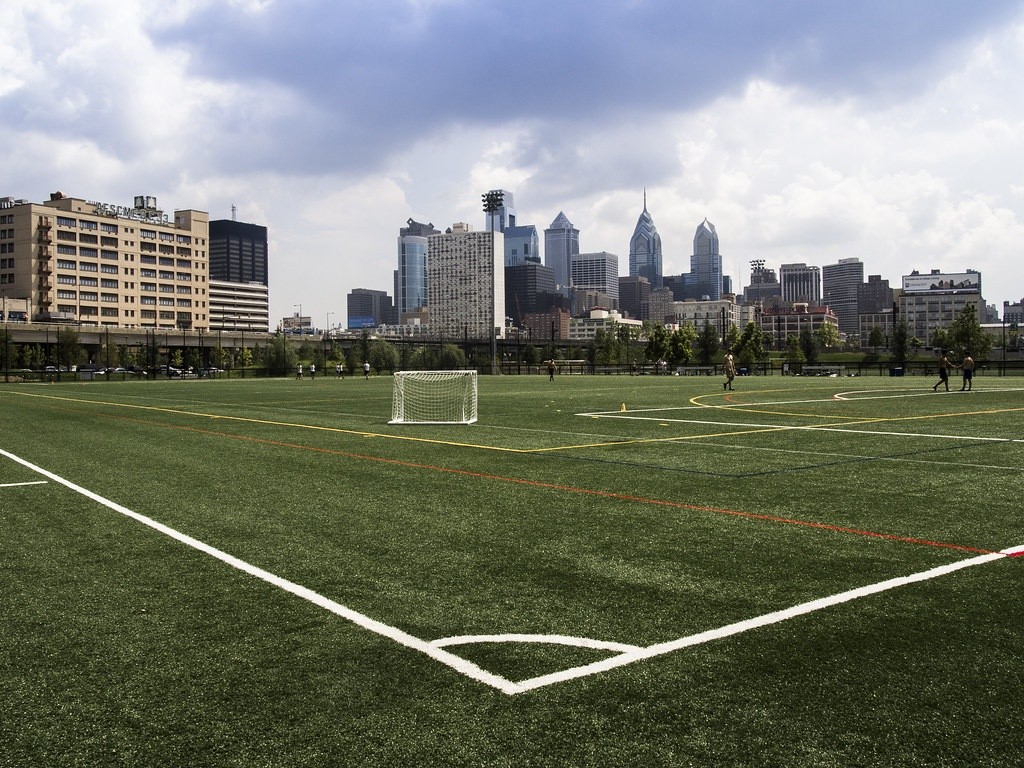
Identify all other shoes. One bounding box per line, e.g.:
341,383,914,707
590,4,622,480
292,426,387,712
724,383,726,389
933,386,936,391
730,388,734,390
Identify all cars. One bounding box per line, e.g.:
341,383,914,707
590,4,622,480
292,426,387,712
46,363,194,377
203,367,225,376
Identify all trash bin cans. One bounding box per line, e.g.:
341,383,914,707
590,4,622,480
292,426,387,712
739,368,747,376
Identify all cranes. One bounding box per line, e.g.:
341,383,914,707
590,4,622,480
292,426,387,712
555,278,610,293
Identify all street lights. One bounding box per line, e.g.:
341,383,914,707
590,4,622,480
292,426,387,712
293,304,302,327
748,259,766,333
38,327,48,367
480,191,505,379
325,312,334,376
95,325,110,381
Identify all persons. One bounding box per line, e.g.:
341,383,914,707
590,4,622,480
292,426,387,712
363,360,371,380
933,350,956,392
957,351,975,391
334,363,345,380
309,362,317,380
631,358,639,376
723,348,736,391
548,359,556,381
660,360,666,377
296,363,304,381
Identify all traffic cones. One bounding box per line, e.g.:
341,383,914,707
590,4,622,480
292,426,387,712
621,403,627,414
